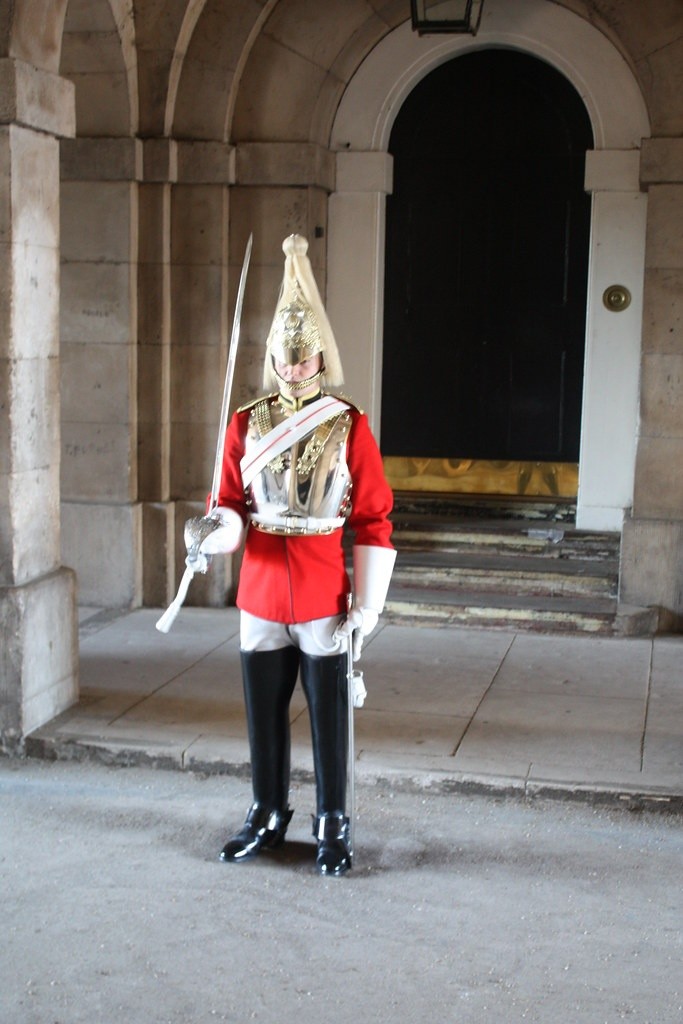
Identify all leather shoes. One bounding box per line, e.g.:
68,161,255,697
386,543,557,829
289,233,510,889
219,801,294,862
310,813,352,875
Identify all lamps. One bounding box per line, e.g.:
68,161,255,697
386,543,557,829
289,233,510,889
411,0,484,37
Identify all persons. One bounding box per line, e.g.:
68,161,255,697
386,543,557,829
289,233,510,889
185,303,398,877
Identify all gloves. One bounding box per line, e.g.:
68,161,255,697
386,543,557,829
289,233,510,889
332,545,398,662
185,507,244,555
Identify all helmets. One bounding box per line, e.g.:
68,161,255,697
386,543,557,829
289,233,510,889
263,233,344,390
